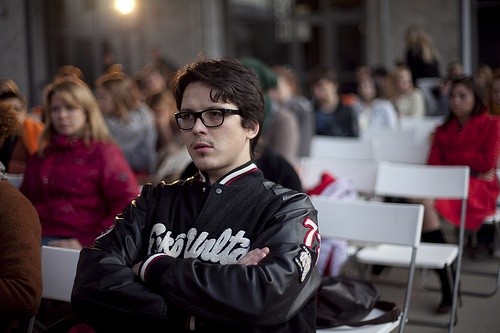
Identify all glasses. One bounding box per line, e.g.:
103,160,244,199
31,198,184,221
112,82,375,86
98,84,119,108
174,108,242,130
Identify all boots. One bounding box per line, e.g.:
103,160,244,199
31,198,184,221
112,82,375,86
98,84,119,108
420,229,462,316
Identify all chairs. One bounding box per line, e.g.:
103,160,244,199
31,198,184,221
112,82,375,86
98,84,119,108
297,98,500,333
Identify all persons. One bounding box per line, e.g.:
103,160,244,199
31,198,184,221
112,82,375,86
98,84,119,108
18,75,140,250
337,25,500,259
70,57,321,333
0,54,180,195
225,60,361,277
370,75,500,315
0,104,42,333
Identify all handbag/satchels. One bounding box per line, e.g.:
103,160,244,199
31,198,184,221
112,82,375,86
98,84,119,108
315,276,402,330
